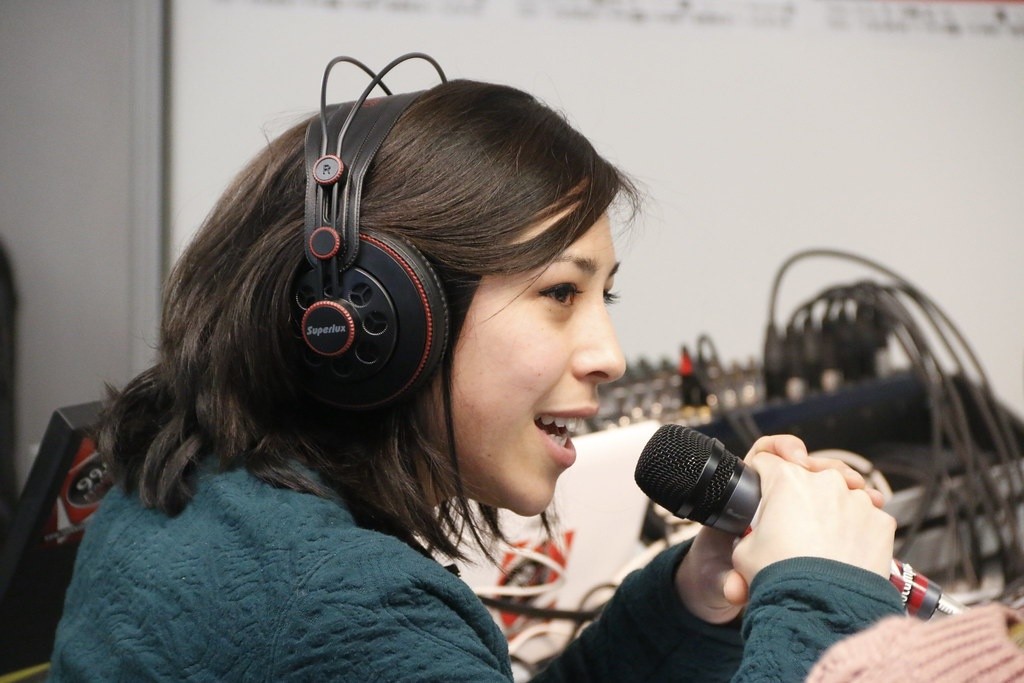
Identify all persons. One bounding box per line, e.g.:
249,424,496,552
49,79,1024,682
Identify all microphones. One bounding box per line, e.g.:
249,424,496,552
635,424,942,622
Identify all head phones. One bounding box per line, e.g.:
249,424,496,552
280,52,454,419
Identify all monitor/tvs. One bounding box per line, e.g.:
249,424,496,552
0,396,122,675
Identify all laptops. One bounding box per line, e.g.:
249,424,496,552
412,419,661,683
